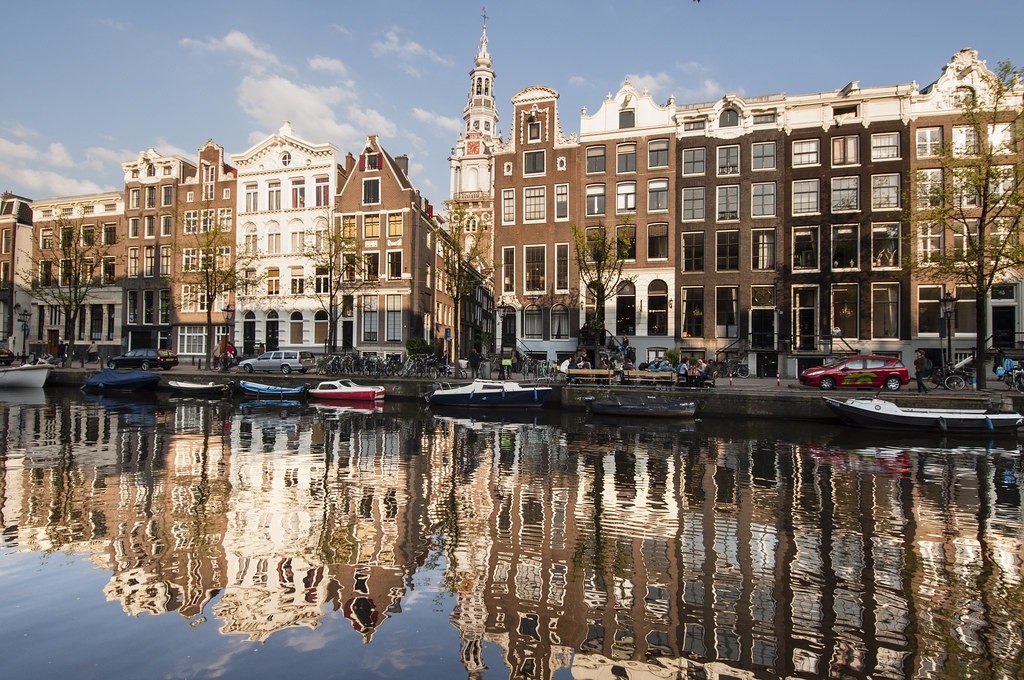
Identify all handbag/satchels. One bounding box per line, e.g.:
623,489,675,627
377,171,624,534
997,366,1004,376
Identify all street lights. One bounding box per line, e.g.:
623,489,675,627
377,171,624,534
18,310,32,365
939,289,957,390
218,309,235,373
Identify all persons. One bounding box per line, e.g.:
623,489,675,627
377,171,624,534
57,341,69,362
645,356,719,388
213,340,237,370
86,341,98,363
914,350,928,394
992,348,1005,381
560,335,635,385
258,343,265,356
441,350,452,377
470,350,480,379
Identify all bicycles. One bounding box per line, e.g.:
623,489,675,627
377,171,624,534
316,352,468,378
928,362,1024,394
716,360,750,379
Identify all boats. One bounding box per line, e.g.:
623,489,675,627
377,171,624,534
422,377,554,407
0,388,550,431
309,378,385,399
239,380,310,396
82,368,161,391
0,357,55,387
168,380,236,393
853,441,1022,486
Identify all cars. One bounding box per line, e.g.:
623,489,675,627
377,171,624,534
107,348,179,371
238,350,316,374
799,355,910,391
0,345,15,366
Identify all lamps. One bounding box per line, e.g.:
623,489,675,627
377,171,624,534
670,298,673,308
581,300,584,310
